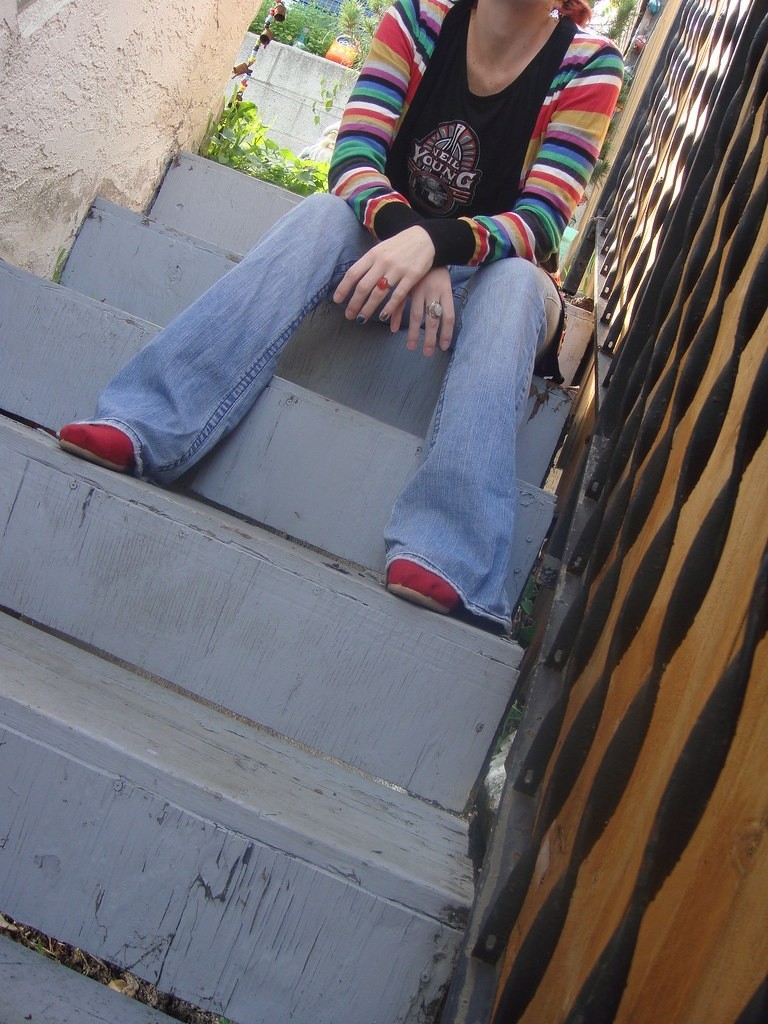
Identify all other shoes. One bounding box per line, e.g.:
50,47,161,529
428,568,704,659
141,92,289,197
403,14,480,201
386,558,459,615
60,422,134,471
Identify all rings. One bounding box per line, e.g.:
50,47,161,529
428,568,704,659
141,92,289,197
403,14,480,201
377,277,392,289
425,302,443,318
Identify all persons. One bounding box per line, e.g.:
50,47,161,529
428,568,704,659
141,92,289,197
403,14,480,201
298,121,342,163
60,0,624,638
